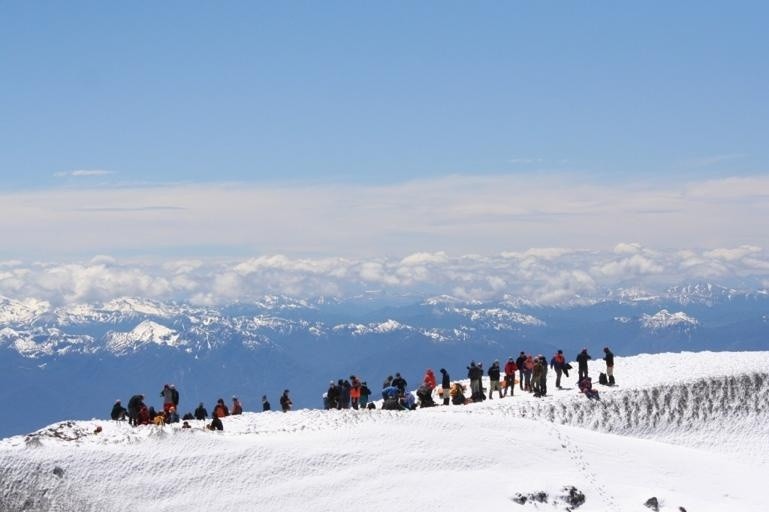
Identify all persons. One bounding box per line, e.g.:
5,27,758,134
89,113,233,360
603,346,615,385
112,384,243,431
280,389,292,412
579,377,592,392
381,372,416,410
262,394,270,411
417,352,546,408
550,350,564,387
322,375,372,410
576,348,591,380
